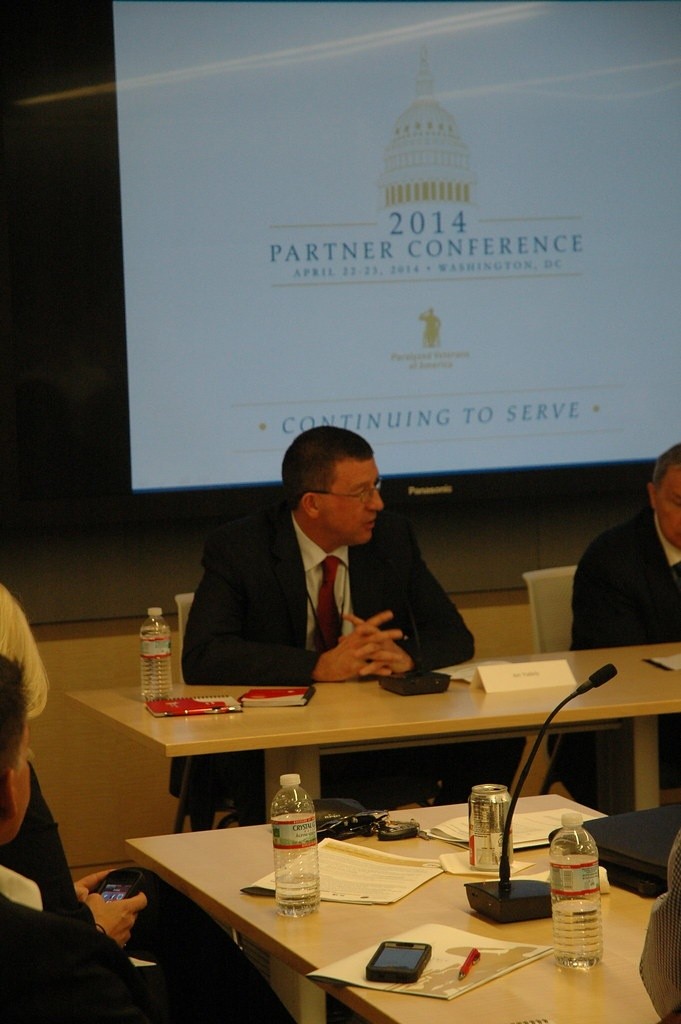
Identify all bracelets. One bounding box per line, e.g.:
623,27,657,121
95,923,106,935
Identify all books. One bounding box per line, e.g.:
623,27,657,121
237,687,316,707
145,694,242,717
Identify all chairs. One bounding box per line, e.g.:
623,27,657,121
171,592,256,833
518,563,575,655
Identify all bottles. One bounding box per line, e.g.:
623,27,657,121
548,812,601,969
139,607,173,702
270,773,320,918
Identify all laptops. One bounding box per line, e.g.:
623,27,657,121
546,801,681,888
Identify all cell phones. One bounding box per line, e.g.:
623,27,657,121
364,942,431,985
97,868,144,905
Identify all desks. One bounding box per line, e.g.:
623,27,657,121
65,642,681,824
123,794,681,1024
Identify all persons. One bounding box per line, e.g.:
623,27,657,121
0,584,295,1024
0,657,146,1024
180,425,524,829
548,443,681,815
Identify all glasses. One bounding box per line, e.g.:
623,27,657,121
317,810,389,837
306,477,382,504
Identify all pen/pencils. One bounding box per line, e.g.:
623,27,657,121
164,706,242,716
458,948,481,980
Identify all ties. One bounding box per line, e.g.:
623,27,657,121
314,556,340,651
674,562,681,595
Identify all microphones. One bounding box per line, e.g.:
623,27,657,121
373,549,452,696
463,664,618,923
